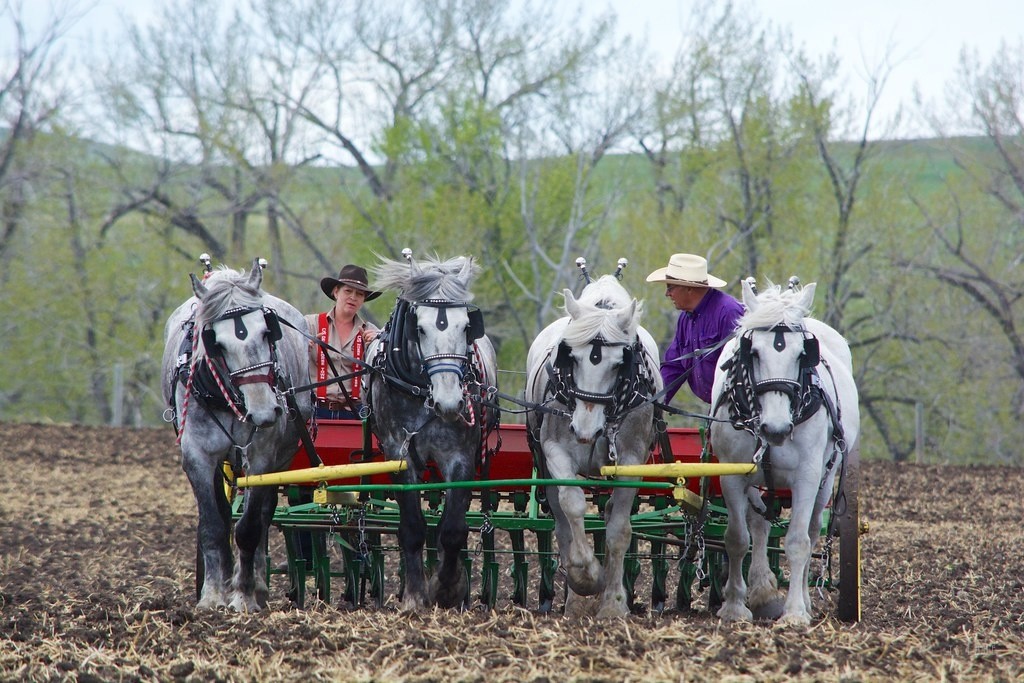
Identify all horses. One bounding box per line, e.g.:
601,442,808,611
524,273,666,624
709,279,861,632
161,257,317,615
359,248,499,620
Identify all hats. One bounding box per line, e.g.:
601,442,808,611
320,264,384,304
645,254,727,287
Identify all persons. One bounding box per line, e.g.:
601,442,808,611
646,253,745,407
278,264,384,575
